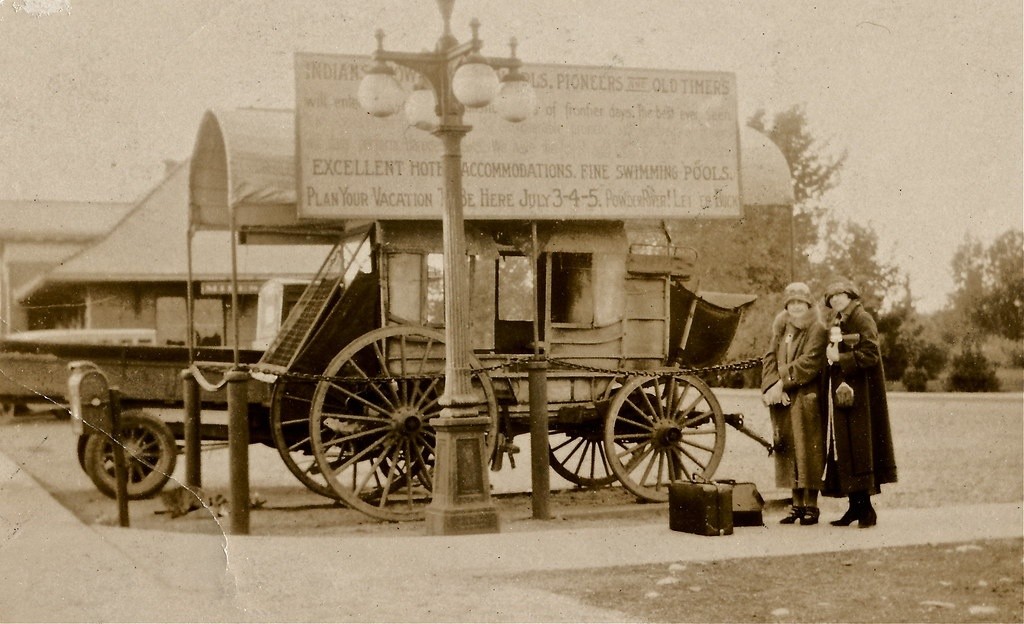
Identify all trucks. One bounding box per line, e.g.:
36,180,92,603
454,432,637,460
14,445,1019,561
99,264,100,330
0,274,344,499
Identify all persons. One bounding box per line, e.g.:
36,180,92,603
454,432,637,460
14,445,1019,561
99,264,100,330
822,276,898,527
762,282,830,525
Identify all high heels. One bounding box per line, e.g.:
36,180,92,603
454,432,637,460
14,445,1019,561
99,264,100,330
801,506,821,525
831,506,860,526
780,505,805,524
859,508,878,528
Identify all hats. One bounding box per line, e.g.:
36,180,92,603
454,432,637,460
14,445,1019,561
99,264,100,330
824,276,861,305
783,283,813,307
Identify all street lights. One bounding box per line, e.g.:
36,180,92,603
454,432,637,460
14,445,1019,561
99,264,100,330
357,0,538,539
